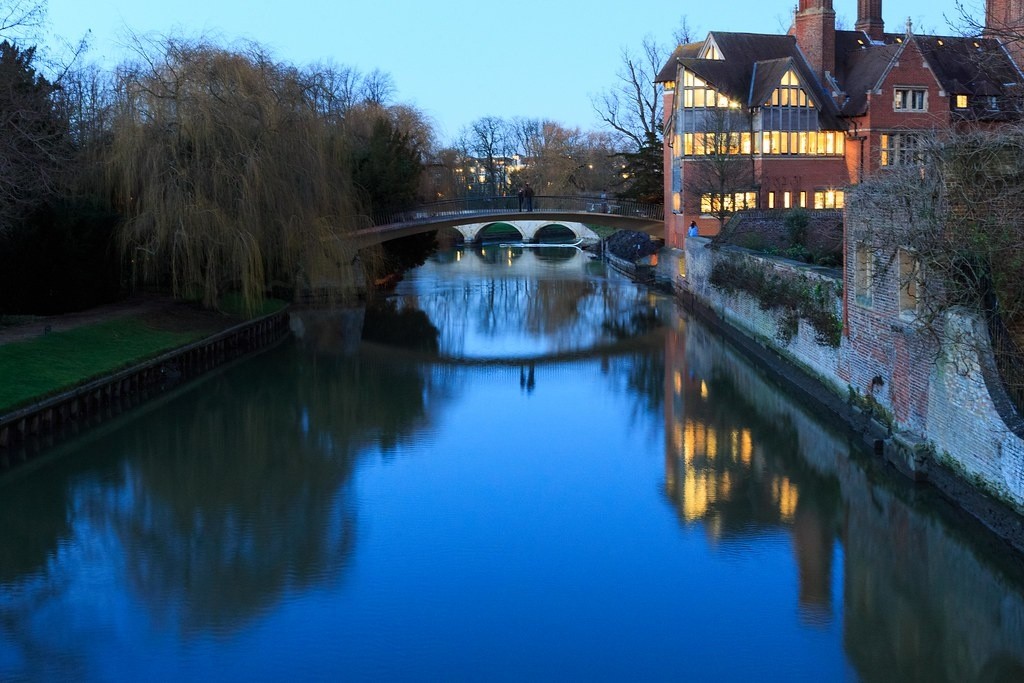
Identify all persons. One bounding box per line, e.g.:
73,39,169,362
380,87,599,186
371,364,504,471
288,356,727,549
518,186,524,202
524,183,534,212
600,189,607,213
687,221,699,238
